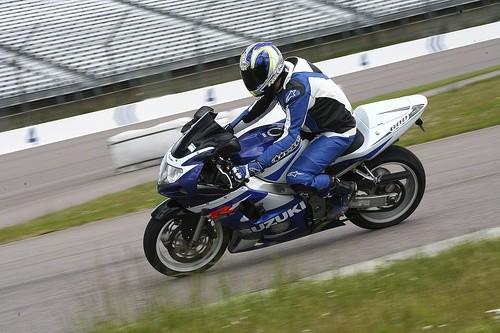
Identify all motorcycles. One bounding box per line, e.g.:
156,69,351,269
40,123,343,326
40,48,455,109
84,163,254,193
142,94,432,279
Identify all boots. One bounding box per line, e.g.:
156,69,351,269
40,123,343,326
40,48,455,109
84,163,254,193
319,174,357,221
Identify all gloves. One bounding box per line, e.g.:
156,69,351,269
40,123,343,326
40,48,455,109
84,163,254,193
229,159,264,184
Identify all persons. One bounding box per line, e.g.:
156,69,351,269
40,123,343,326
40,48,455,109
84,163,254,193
218,41,359,218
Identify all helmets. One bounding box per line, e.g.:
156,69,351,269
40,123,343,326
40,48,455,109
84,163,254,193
239,41,285,97
